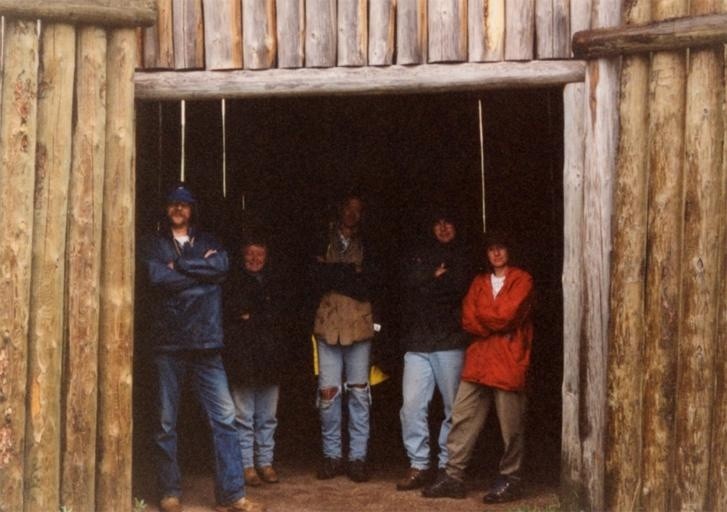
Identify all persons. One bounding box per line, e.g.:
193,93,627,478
395,209,488,490
223,238,291,489
308,194,386,483
422,230,537,503
135,185,264,512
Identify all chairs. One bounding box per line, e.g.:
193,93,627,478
310,334,390,388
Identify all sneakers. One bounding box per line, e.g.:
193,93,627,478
396,465,432,491
158,488,184,511
315,455,370,483
422,476,467,498
218,490,267,512
480,477,526,504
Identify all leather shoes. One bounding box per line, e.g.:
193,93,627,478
244,463,281,487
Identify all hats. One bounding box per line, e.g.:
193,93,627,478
162,183,196,205
